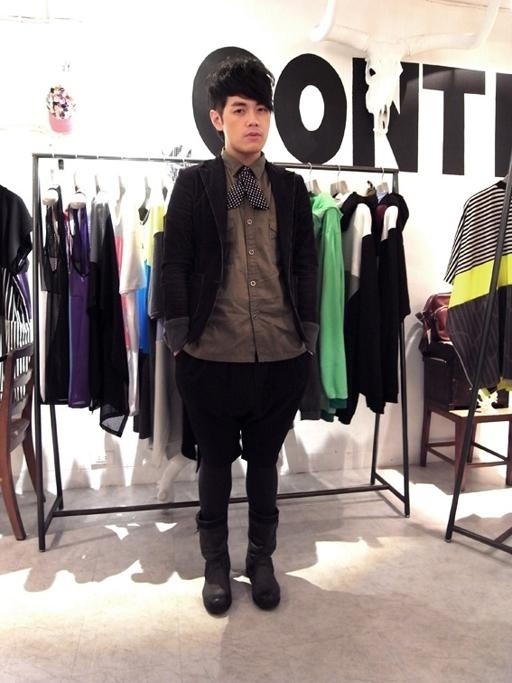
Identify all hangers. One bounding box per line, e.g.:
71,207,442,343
41,148,392,216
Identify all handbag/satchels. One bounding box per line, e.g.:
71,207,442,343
417,293,453,341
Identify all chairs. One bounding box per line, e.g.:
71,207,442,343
421,350,512,493
0,342,46,541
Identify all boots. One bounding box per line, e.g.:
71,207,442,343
246,508,280,609
195,511,231,614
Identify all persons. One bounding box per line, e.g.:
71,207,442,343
154,55,321,617
